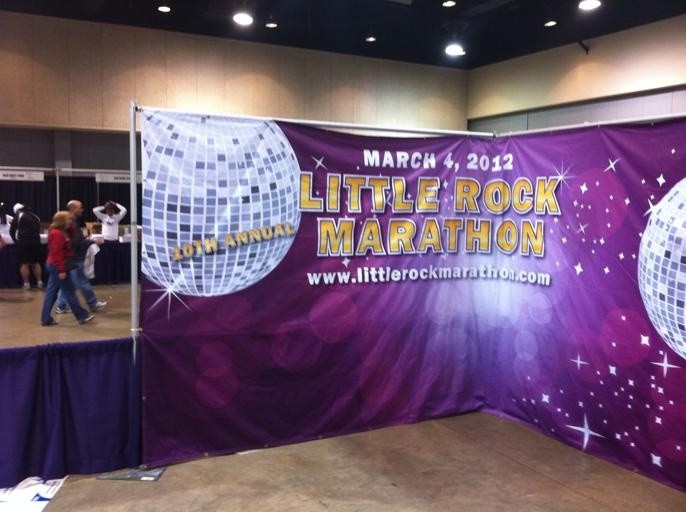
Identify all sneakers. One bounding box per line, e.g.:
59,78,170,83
41,319,59,326
57,304,72,313
23,282,30,290
37,279,44,289
79,313,95,324
91,301,107,312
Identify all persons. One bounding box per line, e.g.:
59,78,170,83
92,200,127,284
9,202,44,290
0,201,18,288
41,211,95,326
55,200,108,314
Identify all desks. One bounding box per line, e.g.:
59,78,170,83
0,234,141,287
